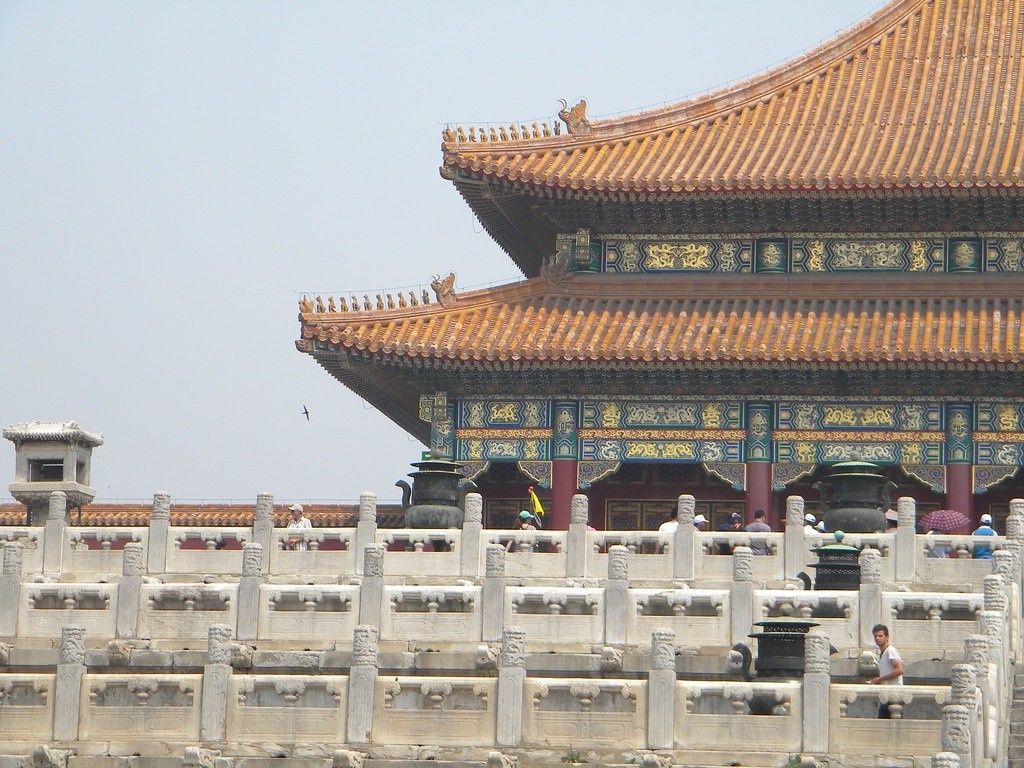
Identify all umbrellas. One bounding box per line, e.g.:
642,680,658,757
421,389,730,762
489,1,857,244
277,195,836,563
877,507,897,520
917,510,971,530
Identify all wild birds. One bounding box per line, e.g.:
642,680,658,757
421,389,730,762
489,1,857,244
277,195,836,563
302,404,310,421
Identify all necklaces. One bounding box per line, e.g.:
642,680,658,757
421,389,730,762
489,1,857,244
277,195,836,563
880,645,888,658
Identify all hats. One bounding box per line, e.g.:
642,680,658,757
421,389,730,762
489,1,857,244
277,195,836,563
981,514,992,523
287,504,303,514
818,521,824,531
730,512,741,519
805,514,816,522
694,515,710,523
519,510,534,519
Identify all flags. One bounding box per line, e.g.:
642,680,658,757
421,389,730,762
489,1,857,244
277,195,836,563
530,493,544,526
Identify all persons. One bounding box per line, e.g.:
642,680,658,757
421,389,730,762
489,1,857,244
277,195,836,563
514,511,537,552
587,520,599,552
802,513,824,533
694,514,721,555
717,512,746,555
282,505,312,551
746,510,771,556
864,624,904,719
926,528,945,558
886,520,897,533
658,508,679,553
972,513,998,559
